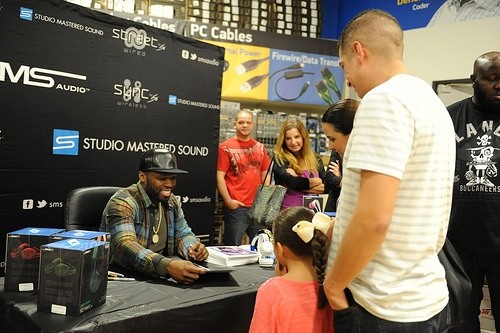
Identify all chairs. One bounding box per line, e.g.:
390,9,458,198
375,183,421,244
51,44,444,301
65,186,122,230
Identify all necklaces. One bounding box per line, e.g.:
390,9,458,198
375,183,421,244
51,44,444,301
151,202,162,244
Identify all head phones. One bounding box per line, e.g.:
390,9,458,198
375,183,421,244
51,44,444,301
11,243,41,260
46,257,76,278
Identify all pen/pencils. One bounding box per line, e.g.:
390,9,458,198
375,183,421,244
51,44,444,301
187,242,199,253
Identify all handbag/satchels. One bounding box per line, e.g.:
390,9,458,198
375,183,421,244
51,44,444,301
248,156,288,227
438,237,472,331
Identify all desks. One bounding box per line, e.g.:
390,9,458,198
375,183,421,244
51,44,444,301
0,262,277,333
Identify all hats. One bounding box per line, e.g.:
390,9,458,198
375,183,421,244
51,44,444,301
139,148,189,174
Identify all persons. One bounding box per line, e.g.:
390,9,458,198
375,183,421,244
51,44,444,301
247,205,334,333
274,118,326,214
324,149,344,213
99,149,209,286
322,98,473,333
426,0,500,27
445,50,500,333
321,8,457,332
215,109,272,246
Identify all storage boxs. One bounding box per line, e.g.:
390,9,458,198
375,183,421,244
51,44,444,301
50,230,111,245
37,239,110,316
4,227,66,294
